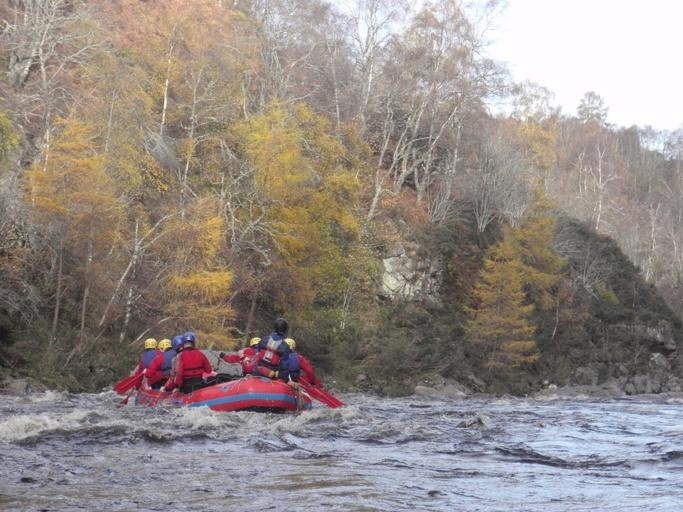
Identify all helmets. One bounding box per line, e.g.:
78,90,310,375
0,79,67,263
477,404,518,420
250,337,261,347
275,320,287,331
144,334,195,352
284,338,296,352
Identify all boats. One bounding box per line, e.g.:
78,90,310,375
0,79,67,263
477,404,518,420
132,350,312,415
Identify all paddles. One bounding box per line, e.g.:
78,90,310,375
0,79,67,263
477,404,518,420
148,391,162,406
140,389,152,404
116,368,143,404
298,376,343,408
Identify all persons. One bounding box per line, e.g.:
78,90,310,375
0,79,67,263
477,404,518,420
130,318,316,395
